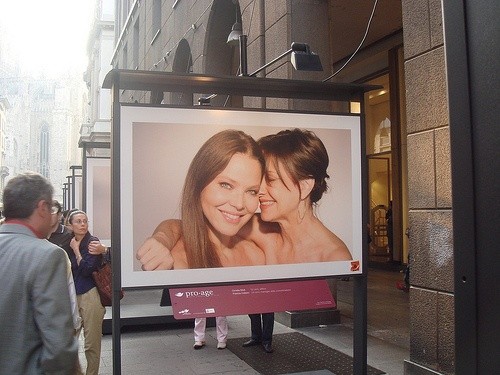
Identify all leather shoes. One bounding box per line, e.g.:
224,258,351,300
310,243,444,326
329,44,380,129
243,339,257,347
263,343,272,353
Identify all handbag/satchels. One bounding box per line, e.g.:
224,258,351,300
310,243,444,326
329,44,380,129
86,237,124,306
395,273,404,290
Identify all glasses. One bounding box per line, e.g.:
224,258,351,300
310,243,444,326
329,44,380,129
46,203,59,214
72,220,89,224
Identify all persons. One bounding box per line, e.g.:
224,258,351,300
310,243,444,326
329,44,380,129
0,172,106,375
193,317,228,349
385,201,393,263
135,127,353,271
243,312,274,353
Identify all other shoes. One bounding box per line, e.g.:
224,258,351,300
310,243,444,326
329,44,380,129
217,341,226,349
193,341,206,349
345,278,349,280
341,278,344,280
